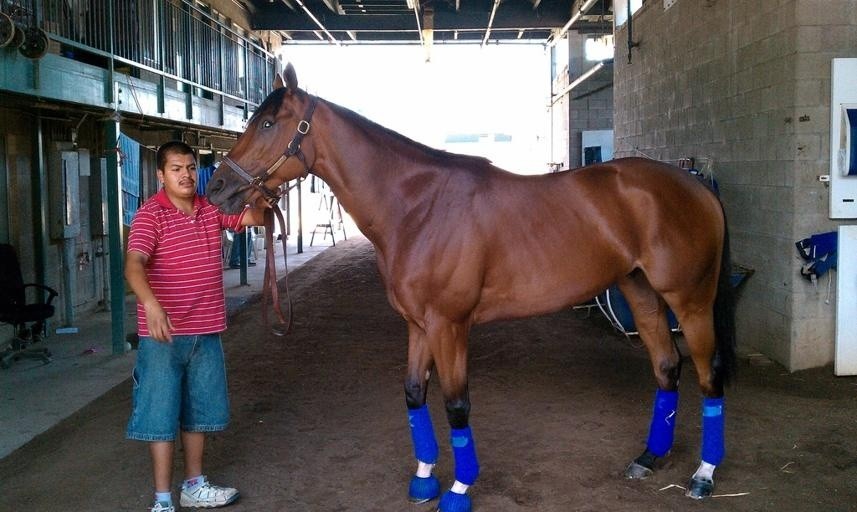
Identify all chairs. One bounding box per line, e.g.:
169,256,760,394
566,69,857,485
0,244,58,369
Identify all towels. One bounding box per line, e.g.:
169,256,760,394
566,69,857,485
120,131,140,227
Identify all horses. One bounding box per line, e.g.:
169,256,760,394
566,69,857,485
203,60,737,512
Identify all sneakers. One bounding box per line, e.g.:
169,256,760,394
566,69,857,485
179,475,240,508
150,499,175,512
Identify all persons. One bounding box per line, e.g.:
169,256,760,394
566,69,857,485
123,141,282,511
228,227,256,269
